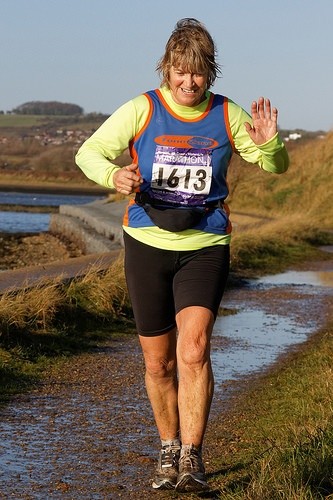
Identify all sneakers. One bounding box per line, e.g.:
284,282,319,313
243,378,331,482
152,444,180,490
176,447,210,493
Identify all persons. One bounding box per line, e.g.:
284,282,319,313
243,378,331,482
75,18,289,493
